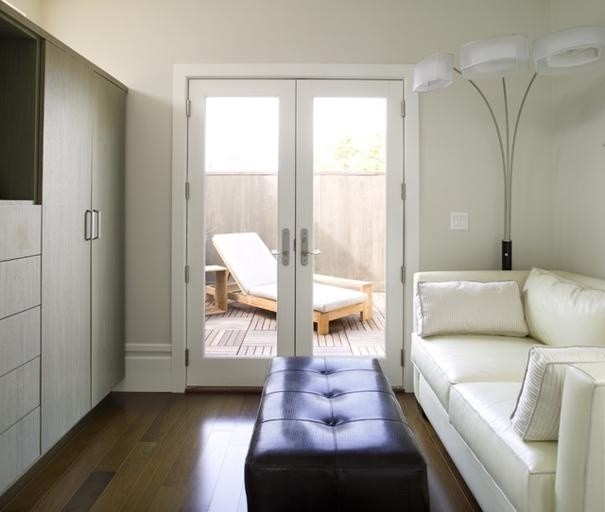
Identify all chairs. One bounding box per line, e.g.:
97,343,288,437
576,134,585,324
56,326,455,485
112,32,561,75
205,231,373,334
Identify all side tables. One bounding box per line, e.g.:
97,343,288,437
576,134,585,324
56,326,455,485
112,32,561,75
205,265,228,316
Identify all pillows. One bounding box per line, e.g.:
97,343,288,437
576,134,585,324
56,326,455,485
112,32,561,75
523,266,604,347
510,344,605,442
414,281,529,339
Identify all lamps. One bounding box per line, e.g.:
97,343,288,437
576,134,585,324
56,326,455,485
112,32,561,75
412,26,605,270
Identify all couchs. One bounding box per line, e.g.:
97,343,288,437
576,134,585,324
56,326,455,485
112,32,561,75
411,270,605,512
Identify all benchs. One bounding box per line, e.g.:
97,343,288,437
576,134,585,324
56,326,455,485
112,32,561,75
244,355,428,512
